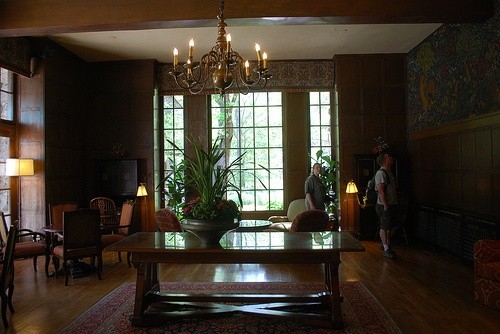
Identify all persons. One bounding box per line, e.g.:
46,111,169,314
374,152,396,259
305,162,326,211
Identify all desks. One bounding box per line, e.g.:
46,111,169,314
105,231,366,329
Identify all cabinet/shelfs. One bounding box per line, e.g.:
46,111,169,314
93,157,138,197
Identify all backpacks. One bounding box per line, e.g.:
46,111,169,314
367,169,390,204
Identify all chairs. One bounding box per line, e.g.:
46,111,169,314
43,202,78,248
51,210,102,286
155,209,184,233
290,209,329,232
0,211,56,334
90,197,135,271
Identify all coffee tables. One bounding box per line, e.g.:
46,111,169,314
234,216,272,232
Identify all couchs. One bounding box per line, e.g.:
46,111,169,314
471,239,500,310
267,198,309,231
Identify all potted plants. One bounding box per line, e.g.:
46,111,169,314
155,131,271,245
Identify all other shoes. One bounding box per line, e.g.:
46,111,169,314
382,248,395,259
378,245,392,251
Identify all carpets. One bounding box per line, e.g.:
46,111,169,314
58,281,403,334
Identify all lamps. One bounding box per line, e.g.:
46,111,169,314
136,181,148,198
6,157,35,243
346,180,359,194
168,0,273,96
